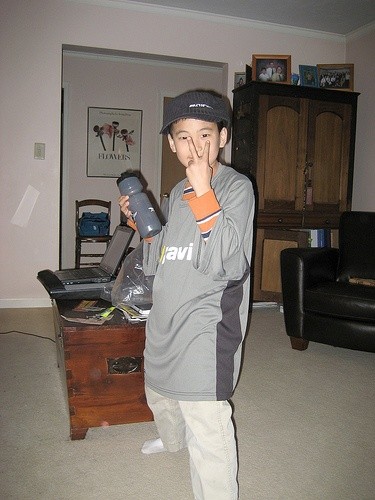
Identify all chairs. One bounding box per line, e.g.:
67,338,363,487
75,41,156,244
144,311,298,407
75,200,112,269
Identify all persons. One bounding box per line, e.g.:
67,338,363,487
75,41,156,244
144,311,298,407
117,89,255,500
306,71,312,85
258,61,286,82
319,71,350,88
237,78,244,87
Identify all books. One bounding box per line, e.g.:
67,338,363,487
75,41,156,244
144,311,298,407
119,303,153,323
61,299,115,325
290,228,339,248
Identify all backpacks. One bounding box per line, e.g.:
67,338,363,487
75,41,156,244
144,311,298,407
78,212,110,237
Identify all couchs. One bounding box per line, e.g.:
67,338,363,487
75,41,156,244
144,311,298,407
280,211,375,351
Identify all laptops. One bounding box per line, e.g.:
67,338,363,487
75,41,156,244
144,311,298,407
55,224,135,285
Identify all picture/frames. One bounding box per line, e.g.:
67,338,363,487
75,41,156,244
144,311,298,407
298,63,354,92
234,54,292,89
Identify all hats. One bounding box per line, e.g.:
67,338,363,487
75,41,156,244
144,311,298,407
159,90,232,134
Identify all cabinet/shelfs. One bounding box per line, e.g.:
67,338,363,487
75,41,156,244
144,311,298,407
52,299,154,440
231,81,361,302
